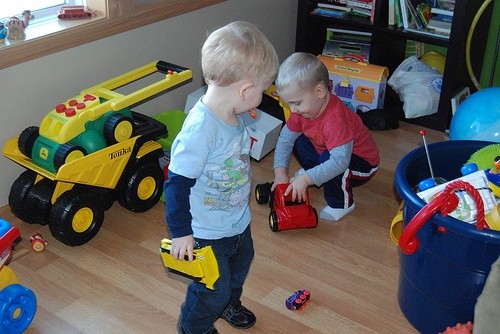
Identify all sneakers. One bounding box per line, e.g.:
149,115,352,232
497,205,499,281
176,320,220,334
220,300,257,329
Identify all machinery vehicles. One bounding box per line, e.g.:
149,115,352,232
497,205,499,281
2,62,196,246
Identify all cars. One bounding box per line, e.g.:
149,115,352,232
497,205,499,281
254,181,320,231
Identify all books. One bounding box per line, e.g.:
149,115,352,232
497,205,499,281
389,0,455,39
311,0,372,19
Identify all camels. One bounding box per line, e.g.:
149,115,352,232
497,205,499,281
5,10,36,40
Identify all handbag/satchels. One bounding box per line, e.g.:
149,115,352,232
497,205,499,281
356,105,399,130
388,56,443,119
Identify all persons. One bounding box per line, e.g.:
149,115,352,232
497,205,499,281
162,20,279,334
271,52,380,222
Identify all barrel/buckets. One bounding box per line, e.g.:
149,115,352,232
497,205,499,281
394,139,500,334
389,199,404,247
